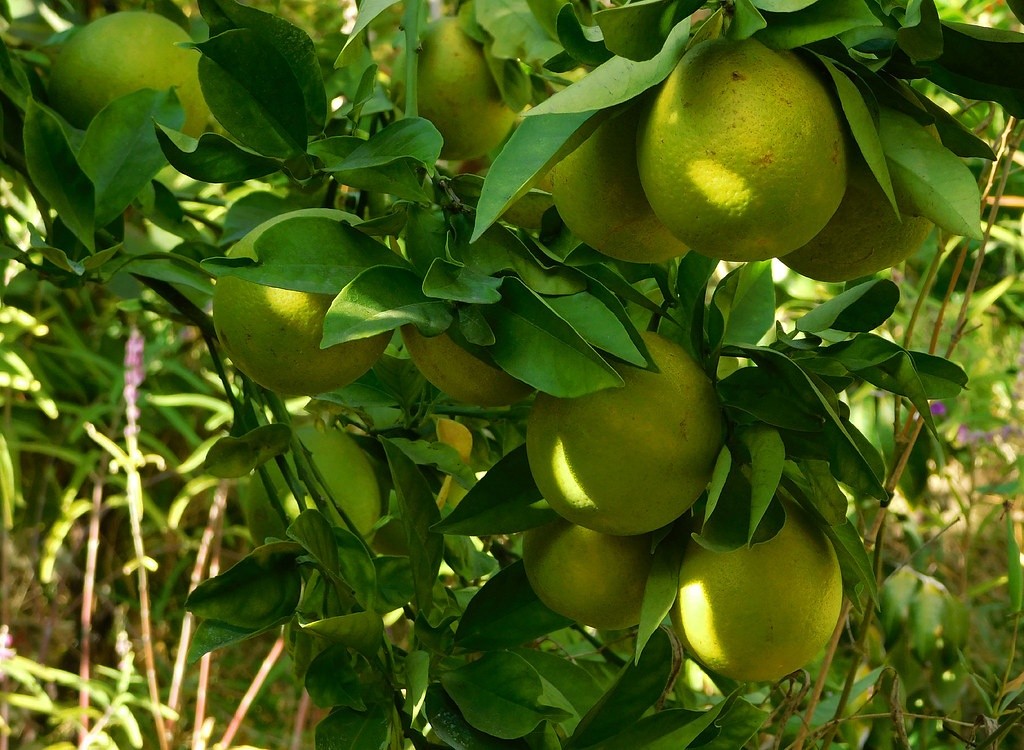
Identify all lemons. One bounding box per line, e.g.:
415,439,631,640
47,8,946,684
